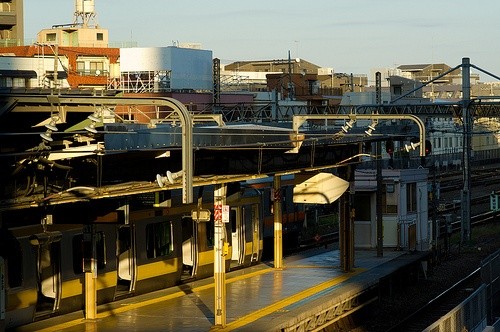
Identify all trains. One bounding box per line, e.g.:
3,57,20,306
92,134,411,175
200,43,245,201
0,180,340,330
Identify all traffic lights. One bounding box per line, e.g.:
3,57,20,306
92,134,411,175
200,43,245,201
424,140,432,155
385,141,394,154
